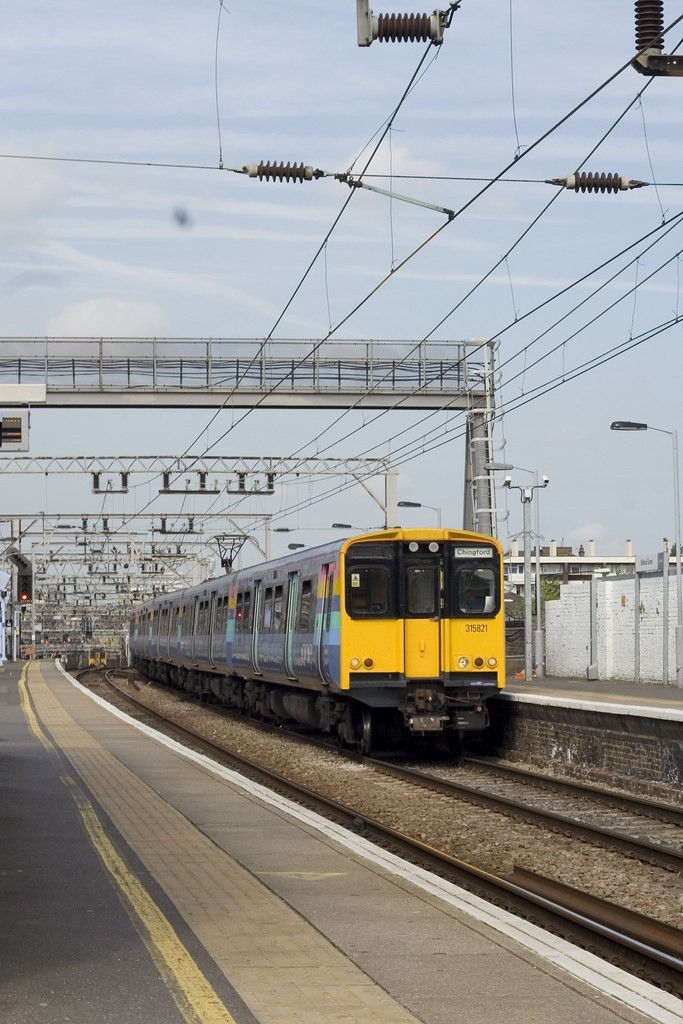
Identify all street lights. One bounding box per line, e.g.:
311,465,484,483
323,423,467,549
482,462,543,680
397,501,442,529
610,420,683,690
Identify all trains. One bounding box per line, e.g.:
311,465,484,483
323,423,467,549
127,529,509,759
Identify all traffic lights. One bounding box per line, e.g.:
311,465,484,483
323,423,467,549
17,575,32,605
